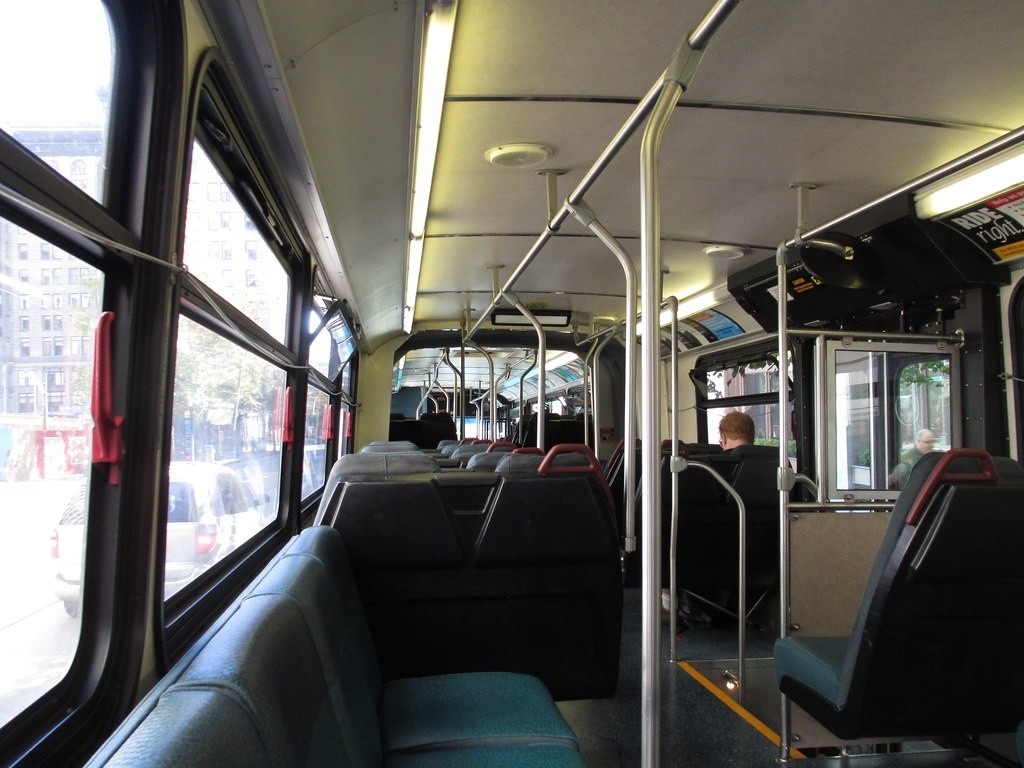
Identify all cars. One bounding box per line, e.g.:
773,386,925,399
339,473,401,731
51,461,272,616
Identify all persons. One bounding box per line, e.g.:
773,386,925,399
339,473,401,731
900,429,935,491
716,413,754,496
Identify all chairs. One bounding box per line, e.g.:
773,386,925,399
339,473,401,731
79,421,1024,768
